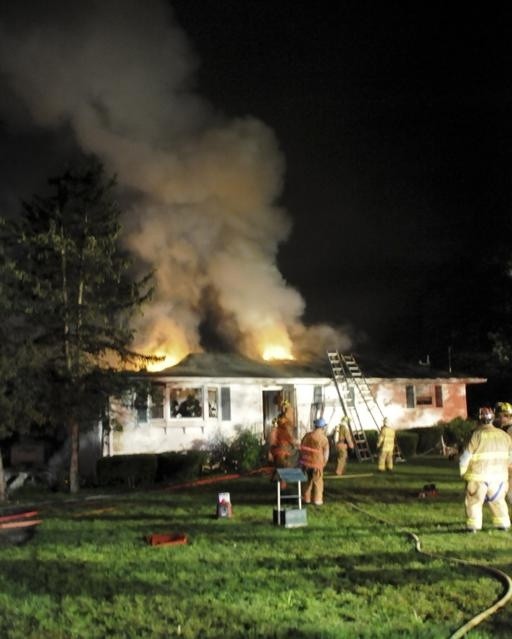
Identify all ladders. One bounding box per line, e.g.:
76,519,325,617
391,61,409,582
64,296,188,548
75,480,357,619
326,348,375,464
340,353,407,464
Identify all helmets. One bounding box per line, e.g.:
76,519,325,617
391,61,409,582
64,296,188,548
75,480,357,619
479,402,512,419
315,418,327,427
383,417,391,427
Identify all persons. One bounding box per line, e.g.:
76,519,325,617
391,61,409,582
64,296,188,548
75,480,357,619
300,417,330,505
456,401,512,530
282,399,295,430
174,393,203,418
375,417,396,472
333,416,354,476
271,416,299,485
